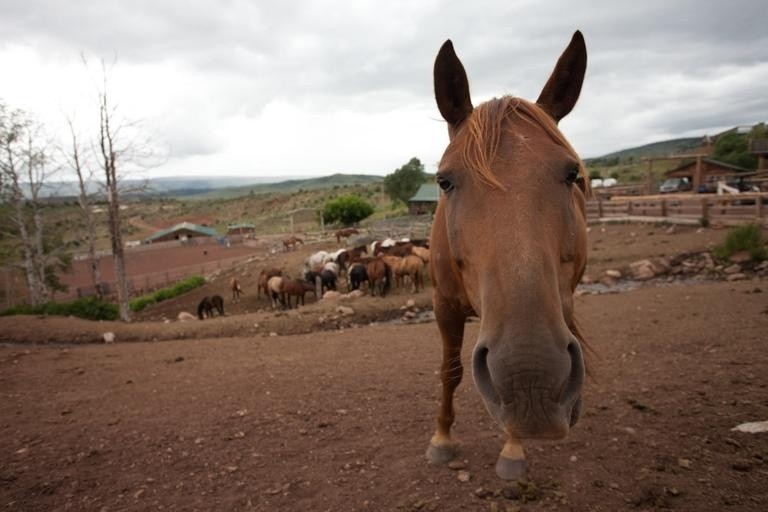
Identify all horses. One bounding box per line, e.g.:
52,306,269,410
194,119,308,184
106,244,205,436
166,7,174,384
423,29,598,484
196,294,224,320
232,278,244,301
255,229,431,310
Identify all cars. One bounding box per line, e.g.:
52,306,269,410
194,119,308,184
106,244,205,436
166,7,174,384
659,177,693,192
